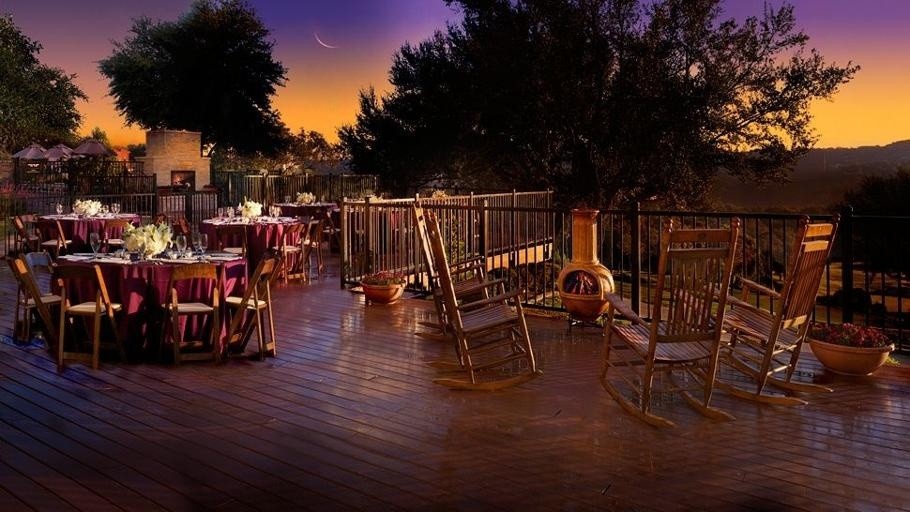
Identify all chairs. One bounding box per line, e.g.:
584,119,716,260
210,258,277,362
421,207,544,391
22,221,56,261
321,208,344,256
685,211,841,408
174,220,201,251
410,193,512,345
103,230,127,252
152,212,172,229
53,263,128,373
13,215,30,254
292,216,326,282
17,255,52,331
271,222,307,288
37,217,72,255
158,260,223,364
7,258,73,347
176,212,209,246
215,224,250,259
597,216,741,431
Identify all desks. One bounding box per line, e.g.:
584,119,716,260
202,216,302,268
274,201,338,216
48,251,250,358
37,213,141,253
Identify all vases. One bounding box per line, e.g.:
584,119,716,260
808,339,896,376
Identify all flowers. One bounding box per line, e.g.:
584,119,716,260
807,321,892,347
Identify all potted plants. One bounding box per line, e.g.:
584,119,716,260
360,270,411,305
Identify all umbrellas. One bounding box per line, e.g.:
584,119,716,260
11,139,118,162
